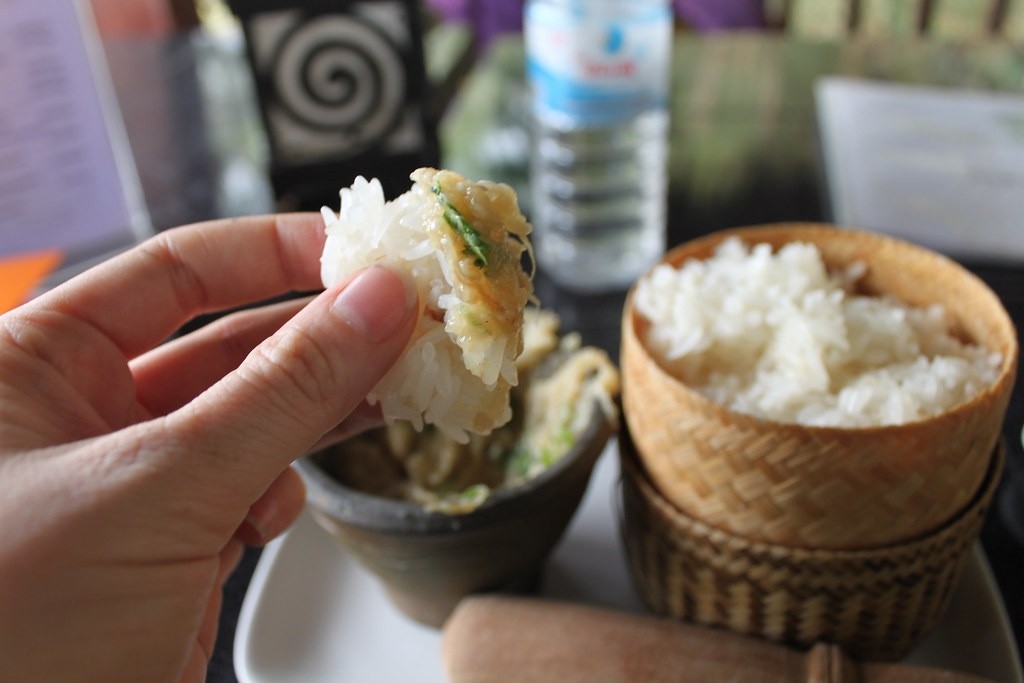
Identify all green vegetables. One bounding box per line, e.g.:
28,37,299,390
431,184,493,271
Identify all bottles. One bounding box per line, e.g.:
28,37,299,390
522,0,666,294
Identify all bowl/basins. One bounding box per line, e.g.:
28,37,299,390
291,342,605,633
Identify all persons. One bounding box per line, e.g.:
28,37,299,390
0,211,420,683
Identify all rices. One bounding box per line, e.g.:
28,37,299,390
632,238,1006,427
317,165,533,442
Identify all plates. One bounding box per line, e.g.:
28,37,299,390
231,435,1023,682
